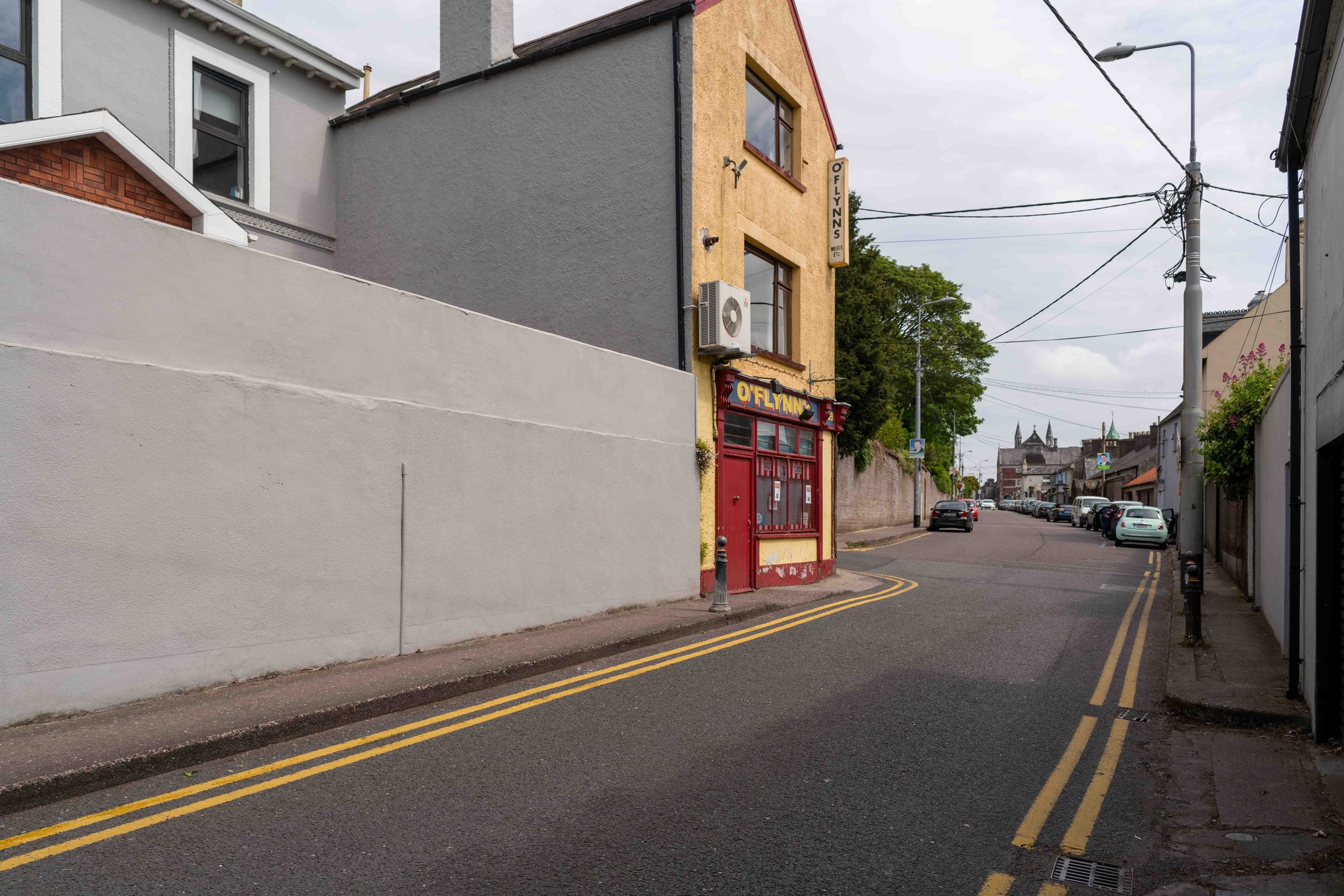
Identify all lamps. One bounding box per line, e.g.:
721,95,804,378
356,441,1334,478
753,376,783,395
799,388,815,421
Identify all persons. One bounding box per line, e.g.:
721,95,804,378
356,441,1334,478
950,469,957,477
1099,504,1118,540
958,477,963,483
913,440,924,453
1099,454,1110,465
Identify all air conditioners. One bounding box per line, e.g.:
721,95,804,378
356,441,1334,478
697,279,751,356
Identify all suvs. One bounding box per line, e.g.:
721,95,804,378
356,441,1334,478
1112,504,1145,542
1109,499,1145,521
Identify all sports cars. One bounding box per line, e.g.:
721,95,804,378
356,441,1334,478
1047,503,1073,522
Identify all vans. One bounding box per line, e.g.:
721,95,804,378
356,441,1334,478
1071,496,1110,528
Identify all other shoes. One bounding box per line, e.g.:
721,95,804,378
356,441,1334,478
1100,536,1106,540
1106,537,1110,540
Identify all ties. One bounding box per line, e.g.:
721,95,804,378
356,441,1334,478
953,474,955,477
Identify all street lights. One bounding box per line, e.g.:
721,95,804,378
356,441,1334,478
914,297,958,528
1091,40,1204,596
961,451,988,507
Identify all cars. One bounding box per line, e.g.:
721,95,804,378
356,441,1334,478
980,499,996,510
1085,501,1115,531
929,500,973,533
1115,506,1176,550
956,498,979,522
997,497,1056,518
